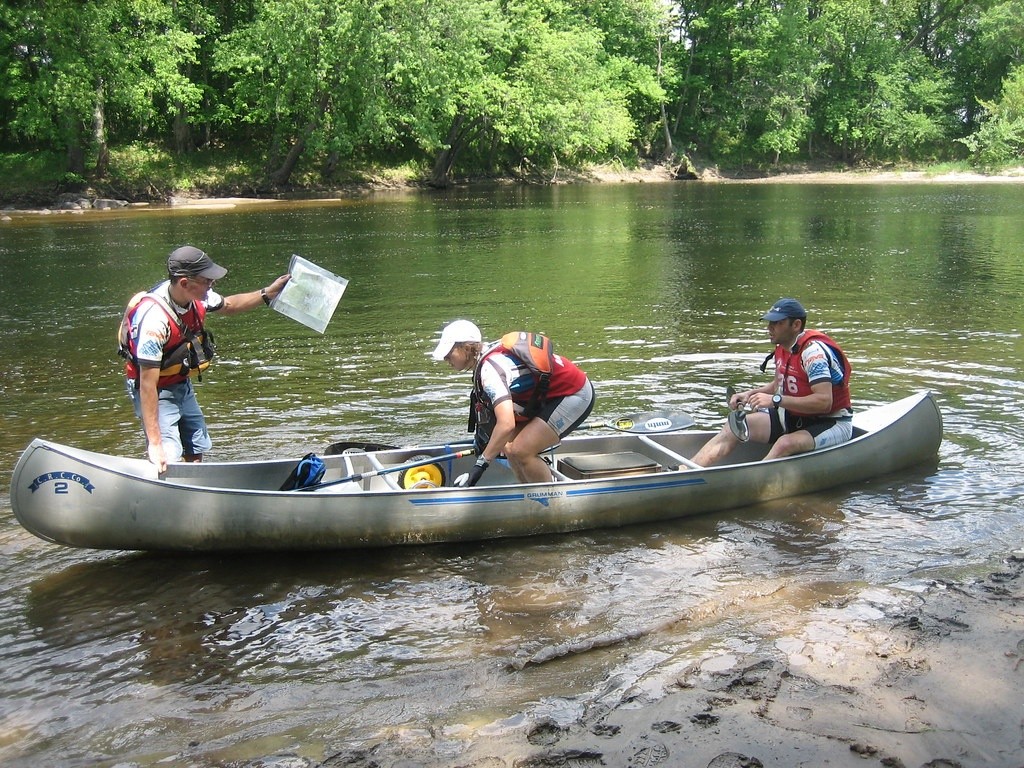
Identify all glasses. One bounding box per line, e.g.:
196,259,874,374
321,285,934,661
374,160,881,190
444,345,457,361
188,275,215,288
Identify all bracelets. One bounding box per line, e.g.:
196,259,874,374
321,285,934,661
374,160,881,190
260,289,270,304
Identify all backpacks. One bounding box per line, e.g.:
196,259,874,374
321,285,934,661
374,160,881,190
279,452,326,491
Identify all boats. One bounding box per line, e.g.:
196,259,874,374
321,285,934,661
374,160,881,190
9,390,946,552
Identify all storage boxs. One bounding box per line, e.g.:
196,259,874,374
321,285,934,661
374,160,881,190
556,453,664,481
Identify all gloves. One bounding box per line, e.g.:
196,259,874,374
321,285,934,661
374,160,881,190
453,455,490,487
472,434,488,458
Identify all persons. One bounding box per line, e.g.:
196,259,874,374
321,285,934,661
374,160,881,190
119,245,292,474
433,320,596,490
662,297,853,471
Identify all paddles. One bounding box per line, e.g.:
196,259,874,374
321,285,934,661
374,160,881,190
725,384,751,443
323,409,698,456
272,437,563,491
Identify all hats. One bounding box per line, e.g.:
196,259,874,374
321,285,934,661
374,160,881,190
759,298,806,321
167,246,227,279
432,320,482,358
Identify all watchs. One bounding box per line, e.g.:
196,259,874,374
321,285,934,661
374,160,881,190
772,394,782,410
478,454,490,465
